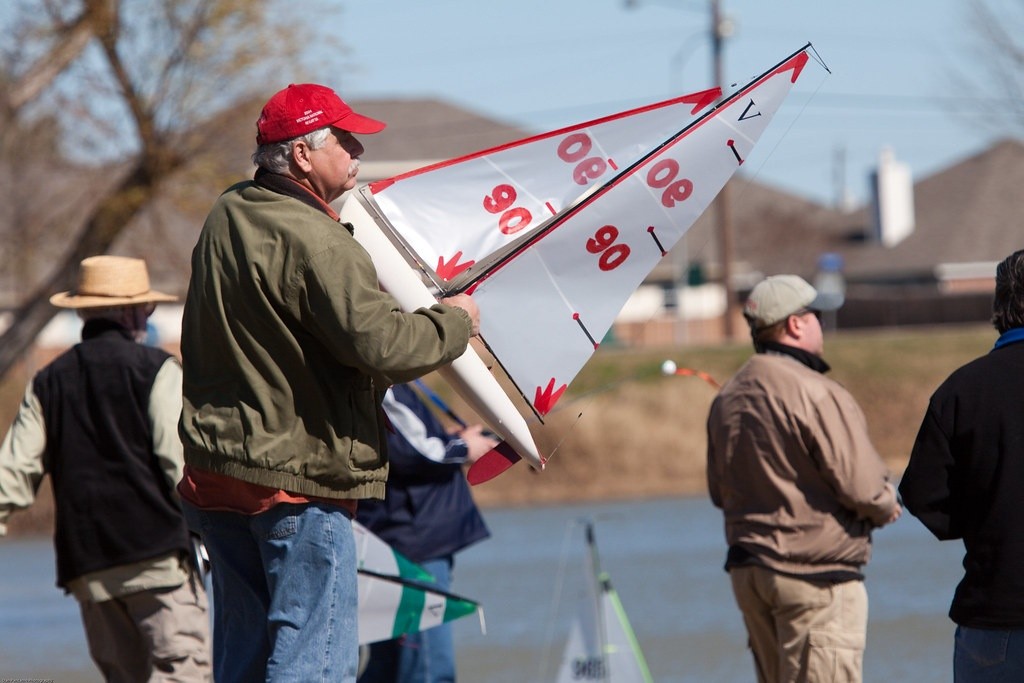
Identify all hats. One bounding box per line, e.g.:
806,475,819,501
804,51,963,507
47,253,180,309
740,273,847,329
253,81,387,146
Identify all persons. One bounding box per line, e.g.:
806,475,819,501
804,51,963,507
352,380,498,683
705,274,902,683
175,82,480,683
898,249,1024,683
0,256,215,682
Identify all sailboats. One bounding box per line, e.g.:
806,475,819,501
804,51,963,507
337,40,831,474
